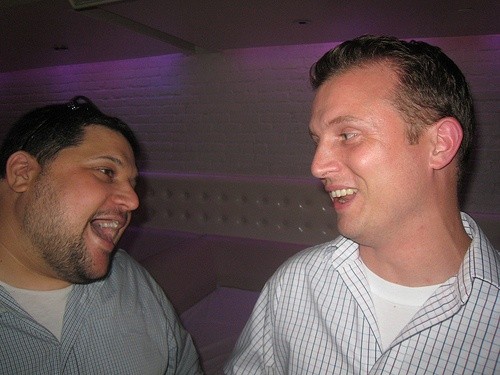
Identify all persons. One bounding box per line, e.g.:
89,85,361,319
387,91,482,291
222,31,500,375
1,93,207,375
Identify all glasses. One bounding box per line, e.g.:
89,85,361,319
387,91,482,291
19,94,101,151
